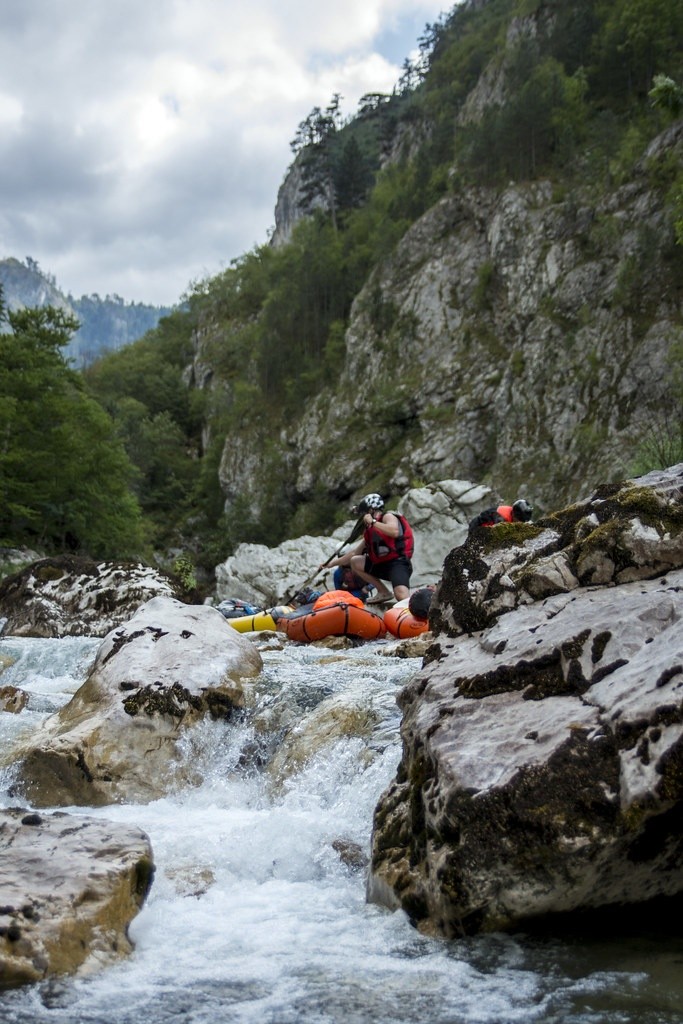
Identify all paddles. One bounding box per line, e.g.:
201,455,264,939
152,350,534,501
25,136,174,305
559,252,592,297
284,514,369,607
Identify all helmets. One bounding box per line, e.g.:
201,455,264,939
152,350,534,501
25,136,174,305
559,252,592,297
359,493,385,512
514,499,533,520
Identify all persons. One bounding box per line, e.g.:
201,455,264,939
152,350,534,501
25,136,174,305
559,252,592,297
319,493,415,603
333,541,371,602
467,500,533,536
